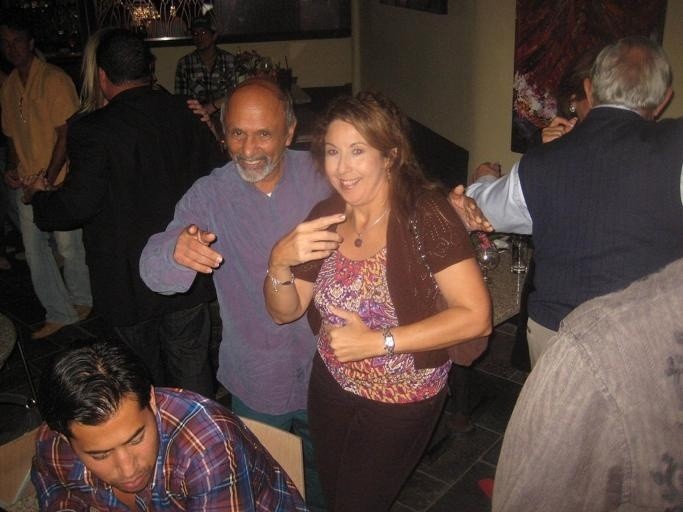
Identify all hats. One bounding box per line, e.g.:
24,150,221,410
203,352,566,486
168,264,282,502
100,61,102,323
188,16,215,33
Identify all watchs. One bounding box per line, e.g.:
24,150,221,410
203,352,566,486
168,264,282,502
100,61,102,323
383,327,397,359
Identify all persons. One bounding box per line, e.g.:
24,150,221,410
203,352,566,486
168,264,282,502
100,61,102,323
1,14,90,341
264,89,489,512
493,262,681,512
30,326,310,510
136,78,490,512
510,61,595,373
24,28,218,395
169,19,240,144
464,37,682,378
36,32,123,182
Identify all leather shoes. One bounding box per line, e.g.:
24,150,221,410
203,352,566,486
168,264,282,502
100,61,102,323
77,305,92,320
32,322,65,339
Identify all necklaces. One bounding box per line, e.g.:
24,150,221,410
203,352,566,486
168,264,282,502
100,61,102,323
345,203,390,249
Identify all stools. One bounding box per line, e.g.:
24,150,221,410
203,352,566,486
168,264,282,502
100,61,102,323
0,314,40,445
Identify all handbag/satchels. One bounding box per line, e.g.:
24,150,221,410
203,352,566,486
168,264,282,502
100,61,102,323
409,186,535,329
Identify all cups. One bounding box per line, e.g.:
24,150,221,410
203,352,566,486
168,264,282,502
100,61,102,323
259,56,275,80
511,237,530,274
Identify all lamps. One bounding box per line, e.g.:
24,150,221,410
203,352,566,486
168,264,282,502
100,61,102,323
139,3,196,44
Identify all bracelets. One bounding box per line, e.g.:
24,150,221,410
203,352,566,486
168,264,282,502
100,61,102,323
266,265,294,291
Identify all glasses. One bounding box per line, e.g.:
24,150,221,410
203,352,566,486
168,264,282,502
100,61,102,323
192,31,210,37
